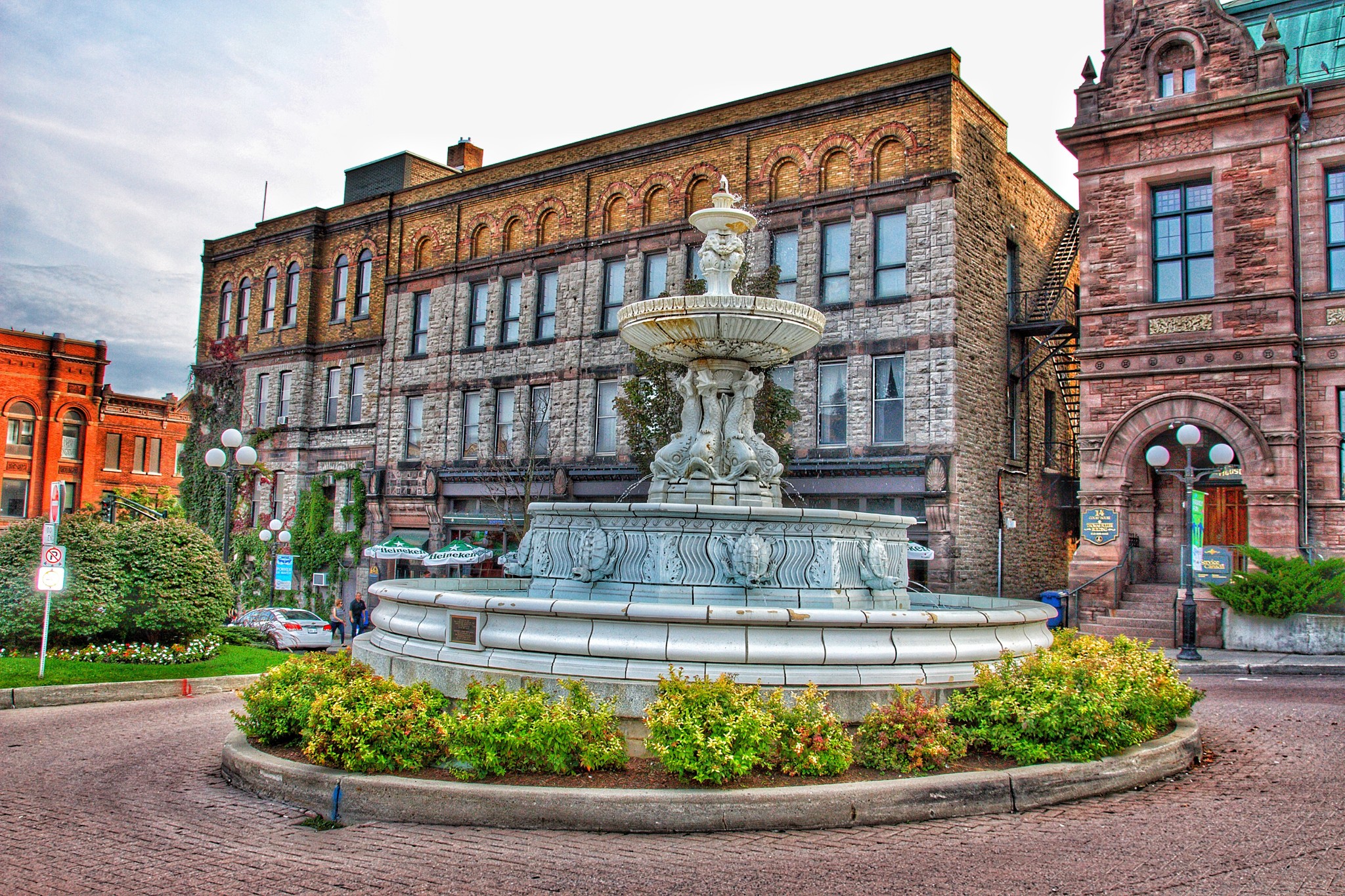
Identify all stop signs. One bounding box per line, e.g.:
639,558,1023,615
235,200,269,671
49,482,59,527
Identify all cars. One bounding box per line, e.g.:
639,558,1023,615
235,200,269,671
227,608,332,652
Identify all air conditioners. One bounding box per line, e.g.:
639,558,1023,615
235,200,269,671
324,476,335,485
277,416,289,425
313,573,330,586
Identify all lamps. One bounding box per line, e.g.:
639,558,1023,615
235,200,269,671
1299,109,1311,133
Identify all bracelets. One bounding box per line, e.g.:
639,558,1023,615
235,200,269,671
344,621,346,623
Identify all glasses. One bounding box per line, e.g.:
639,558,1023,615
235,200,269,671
338,603,343,604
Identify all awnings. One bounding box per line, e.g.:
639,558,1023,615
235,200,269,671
383,529,429,551
443,512,525,527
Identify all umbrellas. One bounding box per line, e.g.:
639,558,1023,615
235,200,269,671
490,542,518,556
497,548,520,564
364,535,429,580
907,540,934,560
421,537,493,566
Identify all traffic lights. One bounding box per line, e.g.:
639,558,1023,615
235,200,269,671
100,499,112,522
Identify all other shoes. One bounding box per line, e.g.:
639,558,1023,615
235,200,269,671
341,644,347,647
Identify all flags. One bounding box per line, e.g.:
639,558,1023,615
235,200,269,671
1191,488,1205,573
274,554,294,591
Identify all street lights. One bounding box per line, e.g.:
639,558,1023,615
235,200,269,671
205,429,257,566
1146,425,1234,663
259,520,292,607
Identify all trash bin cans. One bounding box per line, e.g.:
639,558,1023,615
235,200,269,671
1040,590,1068,629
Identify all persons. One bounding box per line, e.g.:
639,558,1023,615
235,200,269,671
329,598,347,647
423,564,473,578
464,533,474,544
222,606,234,625
349,592,366,641
